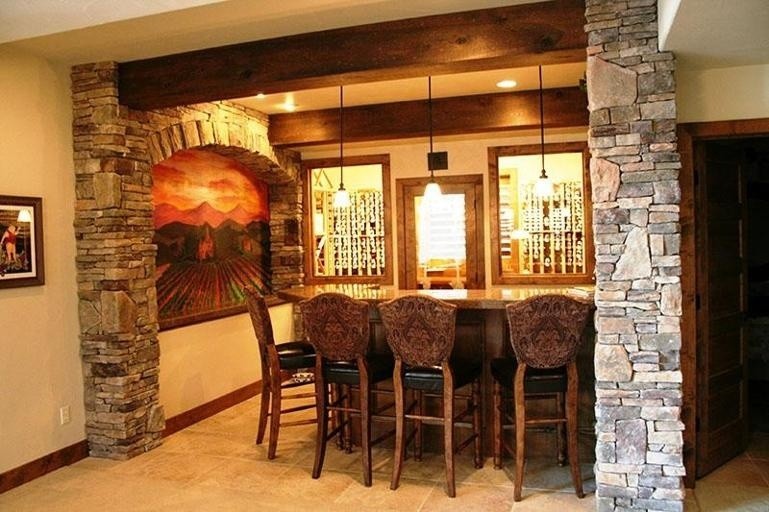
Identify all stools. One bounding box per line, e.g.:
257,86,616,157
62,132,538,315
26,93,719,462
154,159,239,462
298,291,409,488
490,292,592,501
377,295,485,498
243,284,344,462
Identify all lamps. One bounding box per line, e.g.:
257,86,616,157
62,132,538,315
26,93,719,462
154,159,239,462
331,65,556,215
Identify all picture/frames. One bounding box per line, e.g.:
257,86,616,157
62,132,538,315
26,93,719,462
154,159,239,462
1,195,47,290
